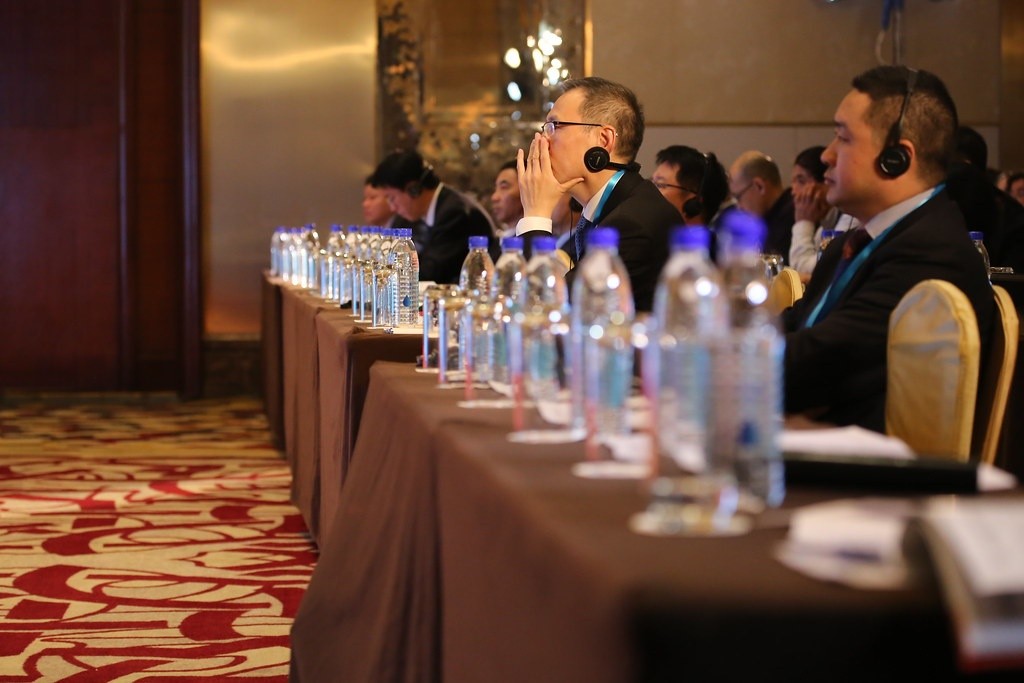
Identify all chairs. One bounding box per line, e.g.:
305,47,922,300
771,268,1019,464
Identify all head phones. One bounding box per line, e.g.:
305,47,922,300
408,165,434,198
584,146,642,173
877,68,921,179
682,152,711,219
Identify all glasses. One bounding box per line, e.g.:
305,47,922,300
732,181,753,200
540,121,603,137
656,183,697,195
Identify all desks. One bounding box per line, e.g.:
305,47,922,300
291,361,1024,683
260,269,440,552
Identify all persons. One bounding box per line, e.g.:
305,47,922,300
646,145,732,264
370,147,503,284
990,171,1023,207
775,63,1006,466
362,170,431,256
729,148,795,274
956,122,1024,493
790,146,862,283
515,76,690,378
491,158,563,246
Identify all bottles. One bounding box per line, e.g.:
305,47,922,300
818,231,845,261
570,228,634,436
491,238,528,387
458,237,495,375
970,232,990,284
709,217,782,512
513,240,568,404
269,224,419,329
653,227,719,476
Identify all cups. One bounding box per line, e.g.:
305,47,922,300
439,299,468,383
422,287,457,368
